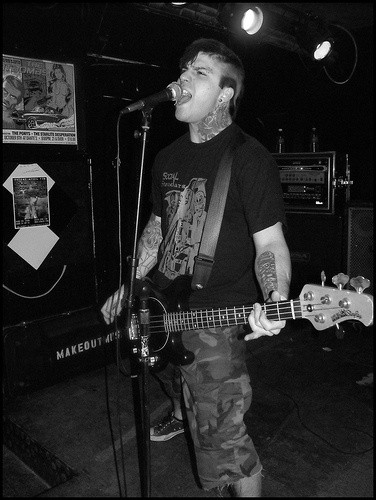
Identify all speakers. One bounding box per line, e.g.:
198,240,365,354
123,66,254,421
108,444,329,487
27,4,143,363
281,212,344,300
343,205,376,298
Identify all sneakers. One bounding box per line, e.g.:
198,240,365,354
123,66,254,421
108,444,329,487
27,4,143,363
150,412,185,442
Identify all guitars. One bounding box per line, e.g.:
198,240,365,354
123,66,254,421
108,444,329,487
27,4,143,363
104,270,373,365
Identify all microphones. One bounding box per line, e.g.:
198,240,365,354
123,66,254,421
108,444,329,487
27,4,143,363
120,82,183,115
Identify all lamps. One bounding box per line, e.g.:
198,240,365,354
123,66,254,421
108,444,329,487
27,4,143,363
217,3,264,35
295,22,333,61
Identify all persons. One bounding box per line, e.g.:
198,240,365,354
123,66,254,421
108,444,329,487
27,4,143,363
47,65,73,113
100,38,291,500
